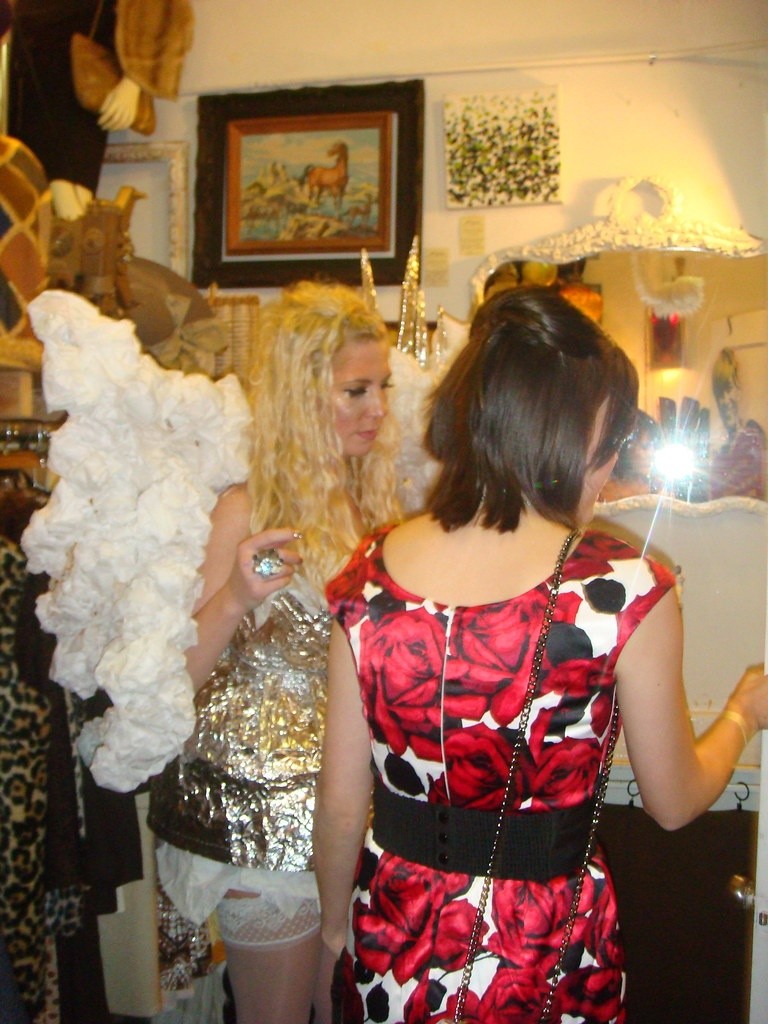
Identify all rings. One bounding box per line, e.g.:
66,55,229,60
253,550,282,575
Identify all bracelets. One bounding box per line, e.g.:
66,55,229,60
719,711,749,747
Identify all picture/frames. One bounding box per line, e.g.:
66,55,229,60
95,143,187,276
467,155,768,520
192,79,424,288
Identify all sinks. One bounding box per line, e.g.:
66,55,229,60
601,711,759,781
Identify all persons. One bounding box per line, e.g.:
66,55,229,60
713,348,764,495
312,287,768,1024
0,0,143,220
599,408,658,502
146,286,392,1024
